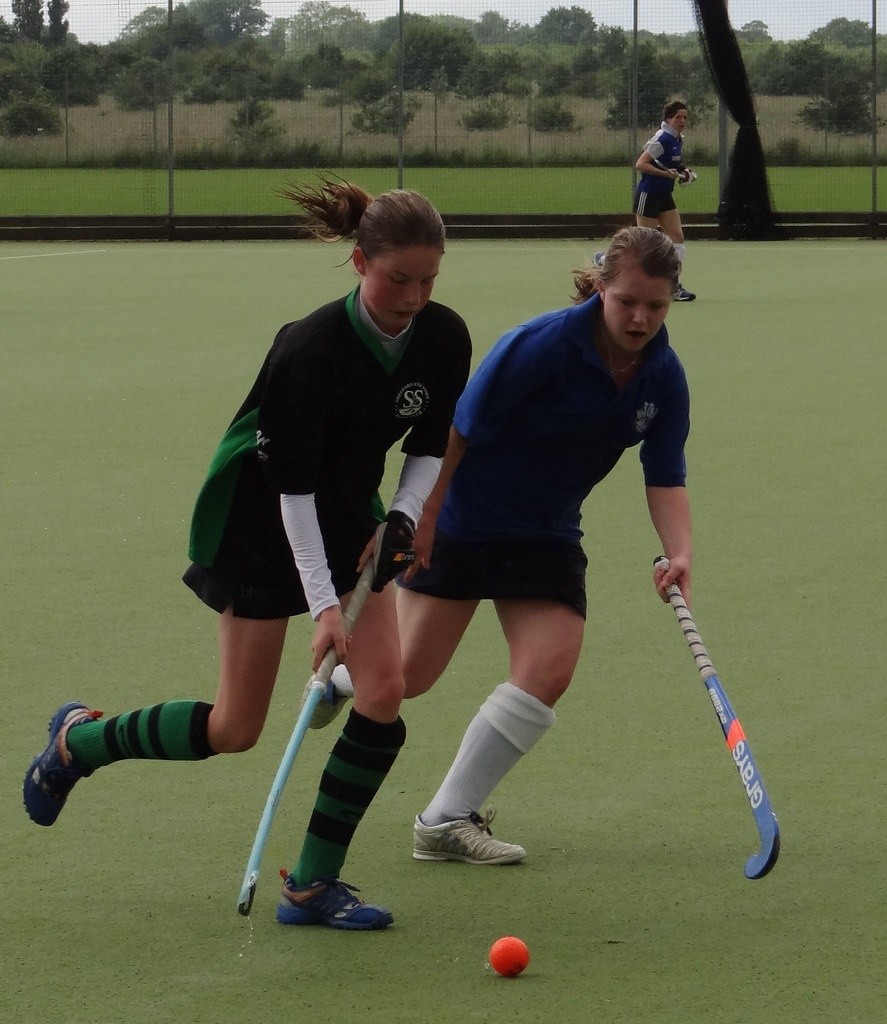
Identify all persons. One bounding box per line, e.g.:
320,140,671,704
592,100,698,302
24,182,474,930
301,225,694,865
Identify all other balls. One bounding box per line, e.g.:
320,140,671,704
488,934,531,982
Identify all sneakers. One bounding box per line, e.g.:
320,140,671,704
276,868,394,930
301,672,351,730
23,701,104,826
672,284,696,302
593,252,605,267
413,808,527,864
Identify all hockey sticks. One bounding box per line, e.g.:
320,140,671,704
653,157,687,179
235,557,374,916
652,556,784,884
